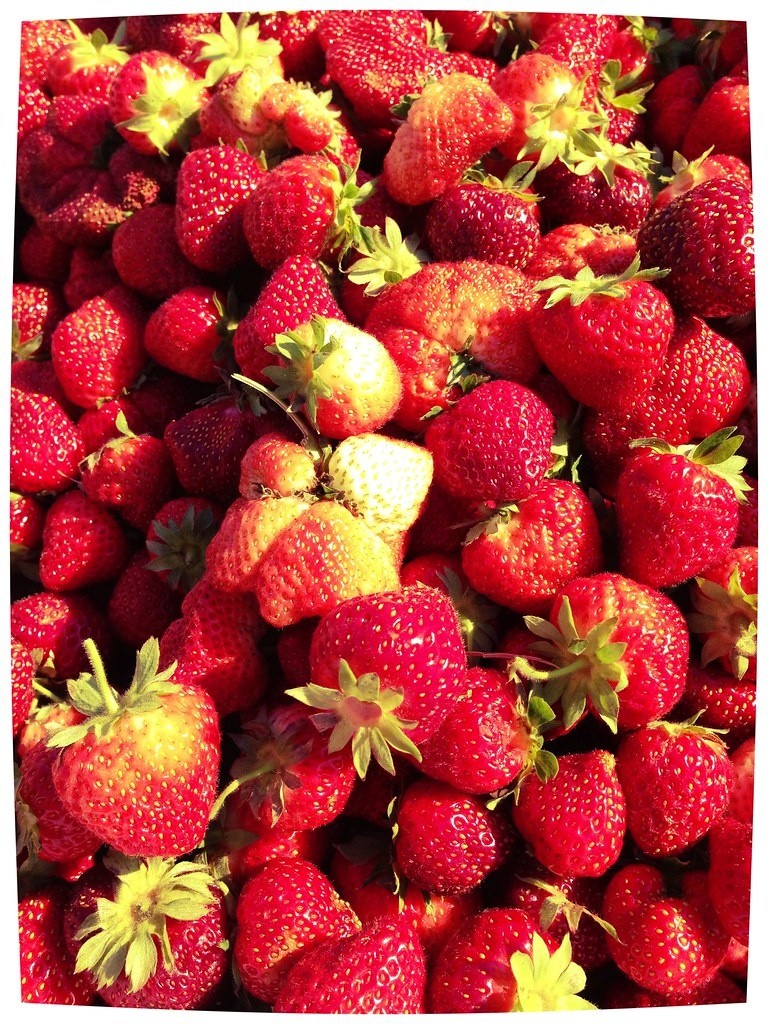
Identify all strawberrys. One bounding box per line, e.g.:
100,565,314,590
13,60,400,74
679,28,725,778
10,10,758,1014
